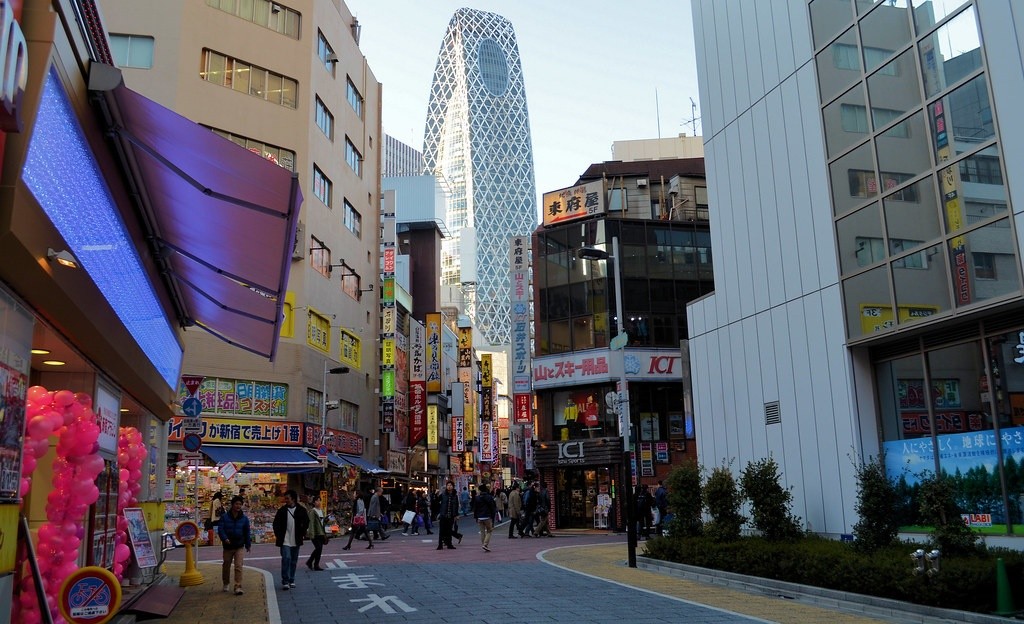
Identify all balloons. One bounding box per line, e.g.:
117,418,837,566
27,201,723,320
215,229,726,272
115,427,148,584
11,386,105,624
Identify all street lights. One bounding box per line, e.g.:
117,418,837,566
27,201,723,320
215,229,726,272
322,359,347,488
576,236,642,568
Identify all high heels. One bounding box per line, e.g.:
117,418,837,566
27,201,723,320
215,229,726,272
342,546,351,550
366,544,374,549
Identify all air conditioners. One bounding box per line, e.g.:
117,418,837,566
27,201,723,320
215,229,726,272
636,179,647,188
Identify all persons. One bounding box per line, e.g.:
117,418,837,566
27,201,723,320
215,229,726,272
211,492,223,526
564,399,578,440
238,488,245,497
634,480,669,541
585,396,598,439
461,487,476,516
342,490,375,551
272,491,310,590
434,481,463,550
491,480,555,539
305,496,329,570
355,487,391,540
402,488,433,536
474,484,497,552
218,496,251,595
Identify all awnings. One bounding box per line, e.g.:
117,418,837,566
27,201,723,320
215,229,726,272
309,451,384,473
200,446,323,473
113,84,303,361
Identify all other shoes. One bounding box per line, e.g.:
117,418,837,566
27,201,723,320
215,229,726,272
284,584,289,588
458,535,463,543
437,546,443,550
306,562,313,571
447,546,456,549
411,533,418,536
509,530,555,539
314,567,324,571
290,583,295,588
482,545,490,551
235,588,243,594
401,532,408,536
224,585,229,592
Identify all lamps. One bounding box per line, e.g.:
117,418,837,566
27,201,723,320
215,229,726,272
291,306,308,311
360,338,380,342
342,268,356,280
329,259,345,272
355,283,373,296
310,241,325,254
315,313,336,319
47,247,81,270
330,325,356,332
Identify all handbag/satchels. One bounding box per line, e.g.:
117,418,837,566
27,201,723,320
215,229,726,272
353,516,365,526
215,506,225,517
368,516,379,531
402,510,416,524
324,535,329,545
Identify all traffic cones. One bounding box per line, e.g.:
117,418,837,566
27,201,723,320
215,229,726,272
992,557,1024,617
